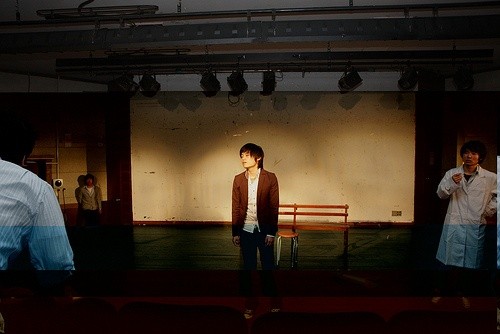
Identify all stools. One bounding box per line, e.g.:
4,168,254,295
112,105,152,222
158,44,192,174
277,231,299,268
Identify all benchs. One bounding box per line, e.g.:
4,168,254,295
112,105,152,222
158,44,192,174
277,204,354,265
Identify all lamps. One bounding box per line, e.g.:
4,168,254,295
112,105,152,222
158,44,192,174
398,71,418,90
119,79,140,97
139,74,160,97
453,69,474,90
338,71,363,90
261,72,277,96
227,72,248,96
200,72,220,91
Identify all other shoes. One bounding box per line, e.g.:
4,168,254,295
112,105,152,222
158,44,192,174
270,306,281,312
461,297,471,310
431,297,442,305
243,308,255,318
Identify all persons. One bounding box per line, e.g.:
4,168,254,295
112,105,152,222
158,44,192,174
231,143,282,320
0,92,81,295
429,140,497,311
77,173,103,226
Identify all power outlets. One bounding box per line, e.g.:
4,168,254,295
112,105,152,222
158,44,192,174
392,211,402,216
